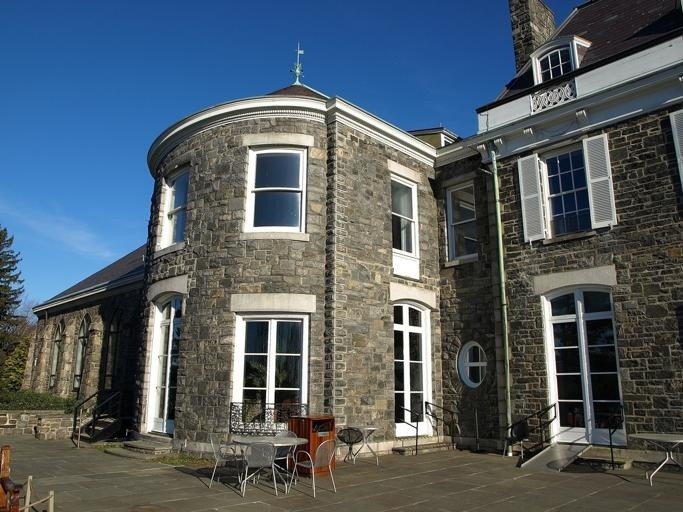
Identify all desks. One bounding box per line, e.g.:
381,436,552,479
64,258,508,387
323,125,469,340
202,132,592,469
348,426,384,466
630,434,683,487
233,436,308,494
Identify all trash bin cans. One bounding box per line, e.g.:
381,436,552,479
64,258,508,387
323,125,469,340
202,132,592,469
288,415,336,479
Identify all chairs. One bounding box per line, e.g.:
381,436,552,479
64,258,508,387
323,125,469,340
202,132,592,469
337,429,363,464
288,439,336,498
210,434,242,488
240,442,278,498
256,431,299,486
